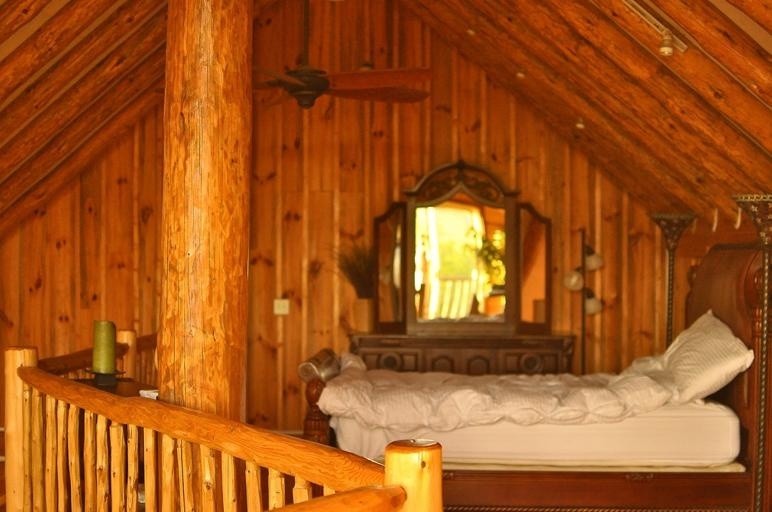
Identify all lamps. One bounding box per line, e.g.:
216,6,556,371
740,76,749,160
566,227,603,374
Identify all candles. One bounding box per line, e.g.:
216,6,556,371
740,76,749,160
91,320,117,373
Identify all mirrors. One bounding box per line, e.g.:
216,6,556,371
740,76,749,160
370,158,550,333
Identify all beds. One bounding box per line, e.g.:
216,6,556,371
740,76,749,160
298,243,772,512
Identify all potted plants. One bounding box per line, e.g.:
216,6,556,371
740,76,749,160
324,241,373,299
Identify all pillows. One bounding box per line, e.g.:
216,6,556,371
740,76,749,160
658,308,756,406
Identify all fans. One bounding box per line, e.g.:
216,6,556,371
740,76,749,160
252,1,434,105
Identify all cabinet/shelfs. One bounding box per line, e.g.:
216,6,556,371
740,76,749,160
350,333,576,375
